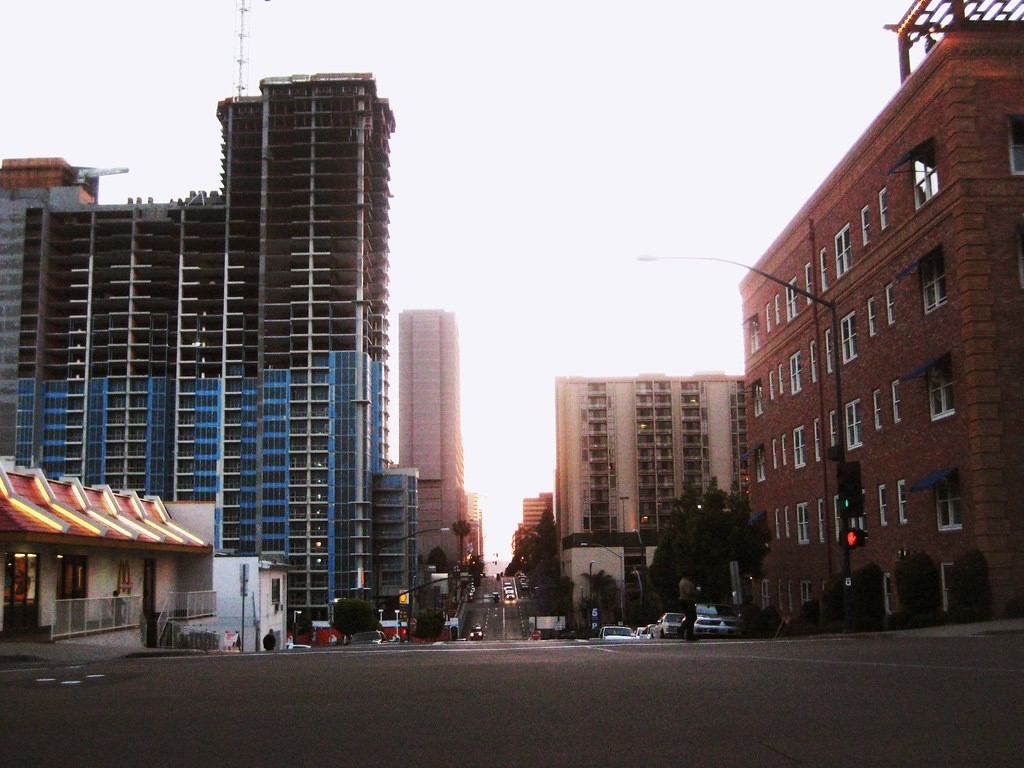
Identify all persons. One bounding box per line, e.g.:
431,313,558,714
677,569,700,641
111,591,126,626
263,629,275,651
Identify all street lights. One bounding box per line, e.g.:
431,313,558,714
589,560,596,638
378,608,383,625
580,543,626,626
293,610,303,645
411,566,435,618
636,254,855,632
376,526,451,619
394,609,400,641
618,496,629,532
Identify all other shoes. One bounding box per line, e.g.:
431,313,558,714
677,628,685,639
686,635,699,641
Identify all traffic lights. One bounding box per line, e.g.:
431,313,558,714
847,525,864,548
836,460,866,519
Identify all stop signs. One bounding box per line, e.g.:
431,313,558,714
532,629,541,640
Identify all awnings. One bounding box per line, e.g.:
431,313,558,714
908,468,956,492
896,243,941,278
748,511,765,521
738,444,764,459
740,313,757,326
742,378,762,392
901,352,949,380
887,137,932,174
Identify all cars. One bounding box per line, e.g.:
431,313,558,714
652,612,687,638
504,572,530,600
597,625,637,639
682,603,749,639
634,623,657,640
469,622,484,641
350,631,387,644
